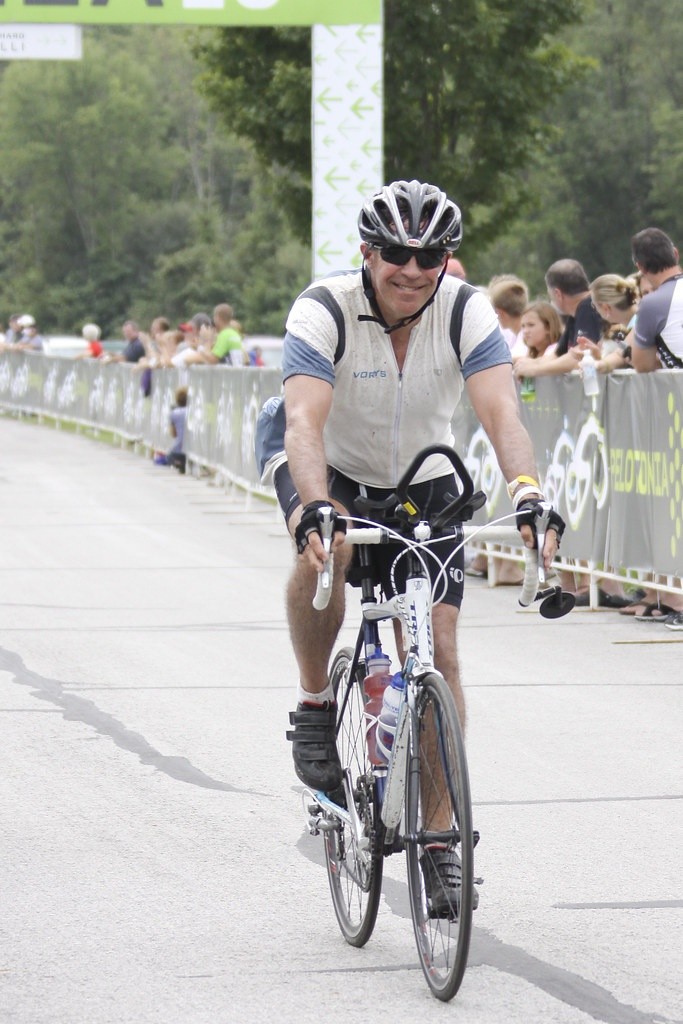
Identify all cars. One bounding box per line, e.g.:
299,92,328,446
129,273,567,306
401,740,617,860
245,335,284,367
43,336,127,362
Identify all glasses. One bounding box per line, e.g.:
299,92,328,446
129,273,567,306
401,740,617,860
591,302,601,310
373,245,447,269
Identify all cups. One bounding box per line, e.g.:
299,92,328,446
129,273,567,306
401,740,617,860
229,349,243,367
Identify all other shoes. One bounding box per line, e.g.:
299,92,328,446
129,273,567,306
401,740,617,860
665,613,683,630
575,593,590,606
599,590,633,607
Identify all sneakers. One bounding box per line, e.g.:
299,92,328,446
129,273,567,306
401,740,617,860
420,851,478,917
287,701,342,791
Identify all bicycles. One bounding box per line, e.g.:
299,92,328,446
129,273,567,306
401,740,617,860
300,444,559,1003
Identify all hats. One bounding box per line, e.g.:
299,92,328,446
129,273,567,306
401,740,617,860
179,322,194,331
18,314,36,325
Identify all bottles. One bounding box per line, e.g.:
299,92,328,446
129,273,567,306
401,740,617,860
375,670,405,764
362,646,392,765
581,349,599,396
519,375,537,402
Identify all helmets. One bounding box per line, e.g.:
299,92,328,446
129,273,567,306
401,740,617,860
358,179,462,250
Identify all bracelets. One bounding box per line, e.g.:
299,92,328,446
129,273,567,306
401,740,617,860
512,487,544,512
507,475,538,502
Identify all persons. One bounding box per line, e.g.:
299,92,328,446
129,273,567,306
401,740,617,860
443,228,683,631
254,179,567,914
0,303,264,471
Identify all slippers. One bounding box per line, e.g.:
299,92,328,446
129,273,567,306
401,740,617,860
465,568,488,579
619,602,654,614
497,579,524,586
635,604,679,621
